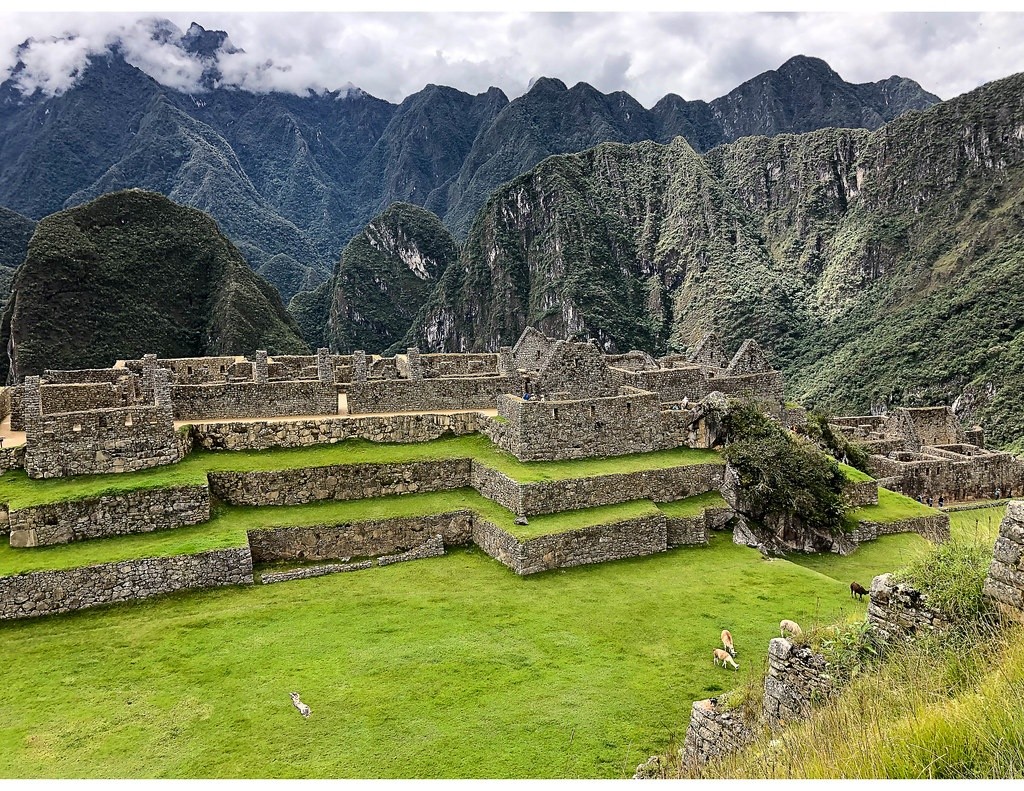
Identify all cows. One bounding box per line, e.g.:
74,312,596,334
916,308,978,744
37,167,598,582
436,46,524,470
712,630,740,670
779,619,802,638
850,582,869,602
